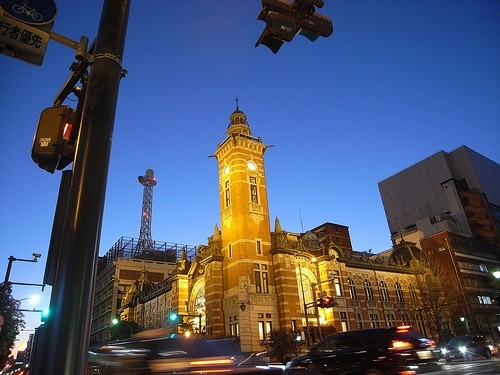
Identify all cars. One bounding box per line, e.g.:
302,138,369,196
86,337,286,375
281,325,444,375
444,335,493,364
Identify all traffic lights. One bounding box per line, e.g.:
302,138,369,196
319,296,334,308
41,309,49,323
254,0,333,55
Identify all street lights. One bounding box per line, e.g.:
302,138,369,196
299,257,316,350
112,318,133,339
